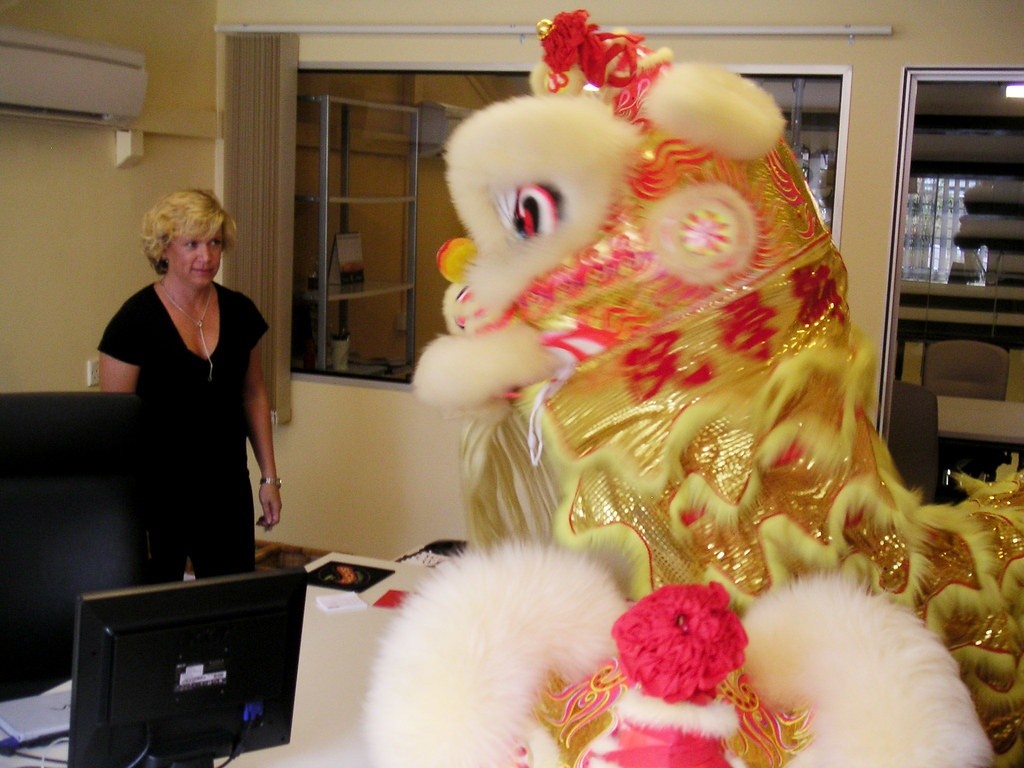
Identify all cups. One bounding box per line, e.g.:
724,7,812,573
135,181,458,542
332,333,349,370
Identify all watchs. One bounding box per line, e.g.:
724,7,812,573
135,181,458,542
260,478,282,489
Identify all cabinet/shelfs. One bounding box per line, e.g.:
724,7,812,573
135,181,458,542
298,93,419,383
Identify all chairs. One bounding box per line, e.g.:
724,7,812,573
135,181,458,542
888,379,937,501
0,390,151,700
924,337,1011,401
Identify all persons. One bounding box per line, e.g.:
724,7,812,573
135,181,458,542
97,188,282,586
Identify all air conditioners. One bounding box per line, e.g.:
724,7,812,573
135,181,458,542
0,25,148,123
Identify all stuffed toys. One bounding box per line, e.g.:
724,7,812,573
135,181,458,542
358,9,1024,768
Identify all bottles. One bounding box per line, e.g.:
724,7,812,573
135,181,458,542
304,337,315,370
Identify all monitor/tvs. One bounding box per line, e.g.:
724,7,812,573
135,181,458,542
67,566,309,768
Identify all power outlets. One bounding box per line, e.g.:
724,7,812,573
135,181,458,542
85,358,99,388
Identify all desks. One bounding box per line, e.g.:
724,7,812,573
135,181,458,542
936,396,1024,493
0,550,430,768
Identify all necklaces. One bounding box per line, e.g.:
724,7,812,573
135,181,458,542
158,278,213,382
